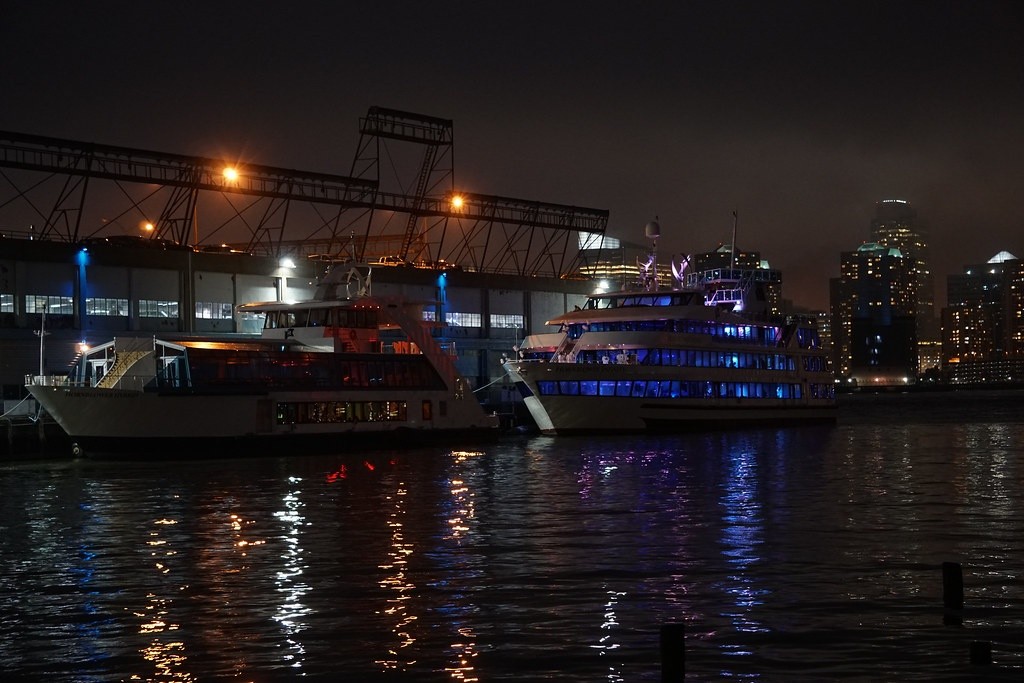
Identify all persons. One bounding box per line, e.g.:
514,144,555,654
602,353,608,364
719,361,734,367
575,305,581,310
761,357,775,369
587,353,593,362
617,353,623,362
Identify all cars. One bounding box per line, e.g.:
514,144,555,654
561,271,590,280
80,234,454,270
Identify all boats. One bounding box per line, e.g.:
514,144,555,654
496,207,841,437
22,229,500,458
